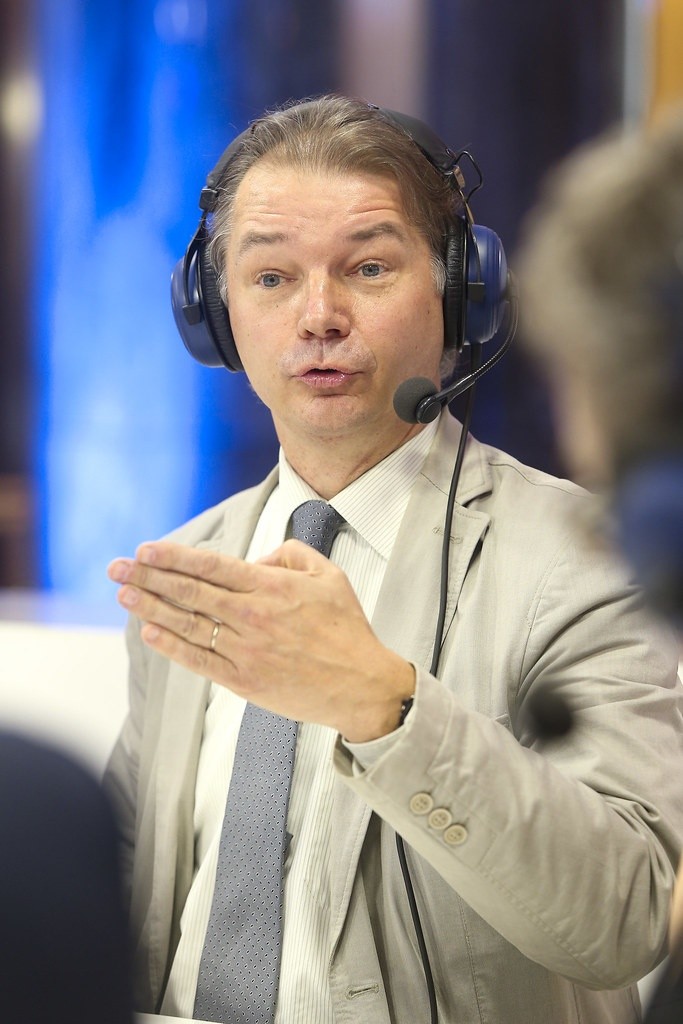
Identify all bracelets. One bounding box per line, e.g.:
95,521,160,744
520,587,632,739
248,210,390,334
398,695,414,726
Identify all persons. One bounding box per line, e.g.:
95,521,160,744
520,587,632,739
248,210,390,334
0,92,682,1024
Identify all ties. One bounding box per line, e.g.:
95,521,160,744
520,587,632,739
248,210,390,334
192,501,344,1024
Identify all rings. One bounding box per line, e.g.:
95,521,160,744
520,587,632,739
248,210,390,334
211,623,218,649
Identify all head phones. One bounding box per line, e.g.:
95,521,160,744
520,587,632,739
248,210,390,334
169,106,511,369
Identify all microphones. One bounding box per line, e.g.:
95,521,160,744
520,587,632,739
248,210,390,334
391,272,520,424
